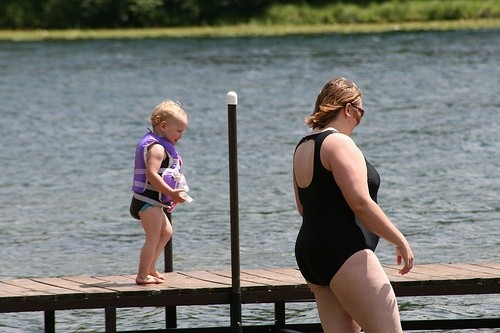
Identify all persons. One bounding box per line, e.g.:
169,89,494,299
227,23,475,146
291,76,415,333
129,99,189,285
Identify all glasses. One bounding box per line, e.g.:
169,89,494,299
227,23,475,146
351,103,364,117
